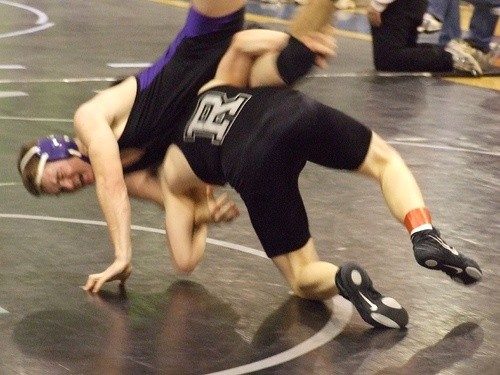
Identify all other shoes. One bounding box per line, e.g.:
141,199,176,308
444,40,482,77
450,40,487,71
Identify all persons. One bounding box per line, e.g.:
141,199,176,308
420,0,500,72
134,29,482,331
17,0,345,293
363,0,489,74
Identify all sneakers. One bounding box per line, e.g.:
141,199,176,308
410,225,483,286
335,262,409,328
